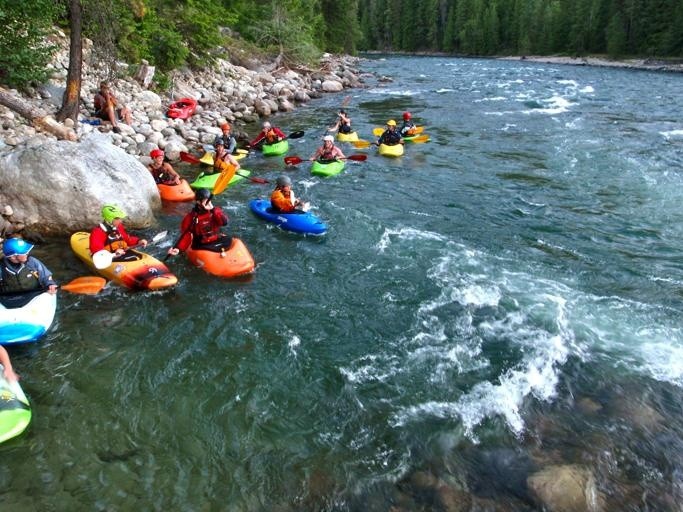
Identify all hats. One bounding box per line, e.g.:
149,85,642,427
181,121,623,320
2,205,13,215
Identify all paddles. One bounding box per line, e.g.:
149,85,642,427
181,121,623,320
0,274,106,297
162,162,238,266
373,126,425,136
91,229,168,270
180,151,268,184
284,154,368,165
240,130,305,151
353,134,429,148
318,95,352,140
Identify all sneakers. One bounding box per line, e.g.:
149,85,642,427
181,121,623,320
114,127,121,132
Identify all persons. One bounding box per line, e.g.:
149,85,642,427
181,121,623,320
166,187,231,257
327,110,350,135
88,206,147,262
212,123,236,155
212,138,240,172
398,112,413,137
145,150,180,185
0,344,20,386
373,120,404,146
309,134,346,162
93,80,130,133
269,175,304,214
0,237,58,296
244,121,286,148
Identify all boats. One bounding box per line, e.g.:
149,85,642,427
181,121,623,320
261,139,289,156
378,142,404,158
180,211,255,279
0,355,32,447
189,167,251,192
396,125,425,141
311,154,346,178
156,179,196,202
165,97,197,120
337,131,360,144
70,231,178,290
248,198,329,237
198,148,249,168
0,242,59,349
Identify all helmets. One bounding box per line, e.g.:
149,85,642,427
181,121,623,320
222,124,231,130
323,135,334,145
150,150,164,157
215,139,225,146
3,237,34,257
102,205,126,225
403,112,411,120
386,120,397,126
261,121,271,129
196,187,209,201
276,175,292,187
340,109,347,116
